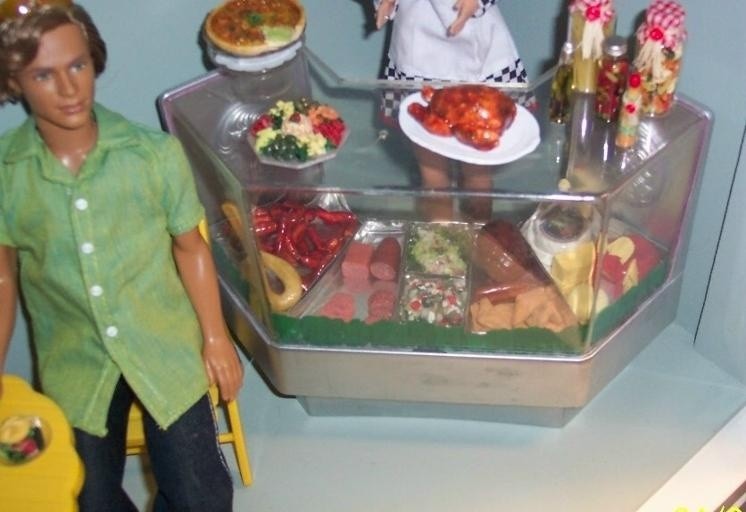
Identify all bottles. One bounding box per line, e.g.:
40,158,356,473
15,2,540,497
566,1,617,93
635,1,689,120
594,37,627,121
547,42,577,125
613,75,642,150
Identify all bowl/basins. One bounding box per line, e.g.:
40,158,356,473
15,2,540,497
205,2,306,57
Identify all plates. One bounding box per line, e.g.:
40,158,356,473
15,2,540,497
398,89,542,167
247,111,350,170
